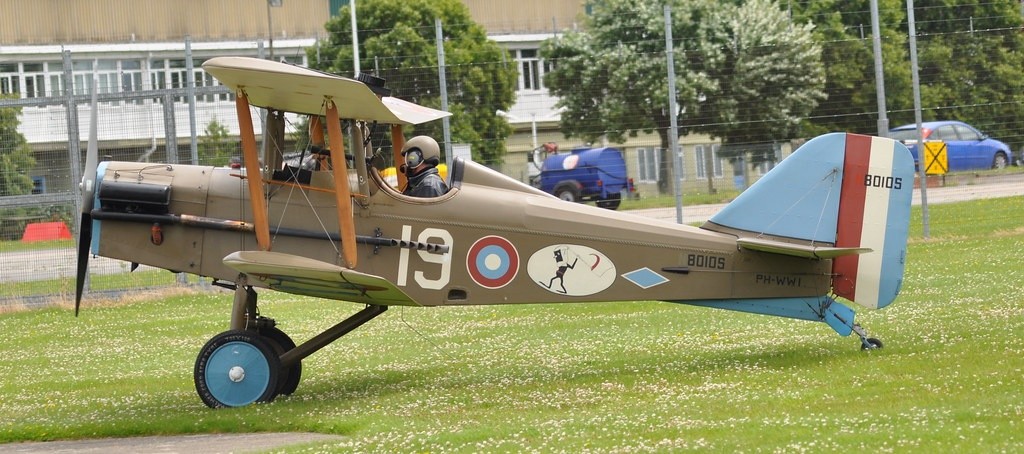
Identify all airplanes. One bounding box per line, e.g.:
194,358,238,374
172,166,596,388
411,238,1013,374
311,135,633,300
75,57,915,409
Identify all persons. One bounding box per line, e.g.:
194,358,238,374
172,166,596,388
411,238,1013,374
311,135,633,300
399,135,448,198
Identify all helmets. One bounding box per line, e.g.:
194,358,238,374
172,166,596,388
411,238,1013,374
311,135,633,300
401,135,440,166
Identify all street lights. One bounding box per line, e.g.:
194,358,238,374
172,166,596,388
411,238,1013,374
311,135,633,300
267,0,282,60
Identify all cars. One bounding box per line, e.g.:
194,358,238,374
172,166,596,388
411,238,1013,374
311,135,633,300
888,121,1013,172
